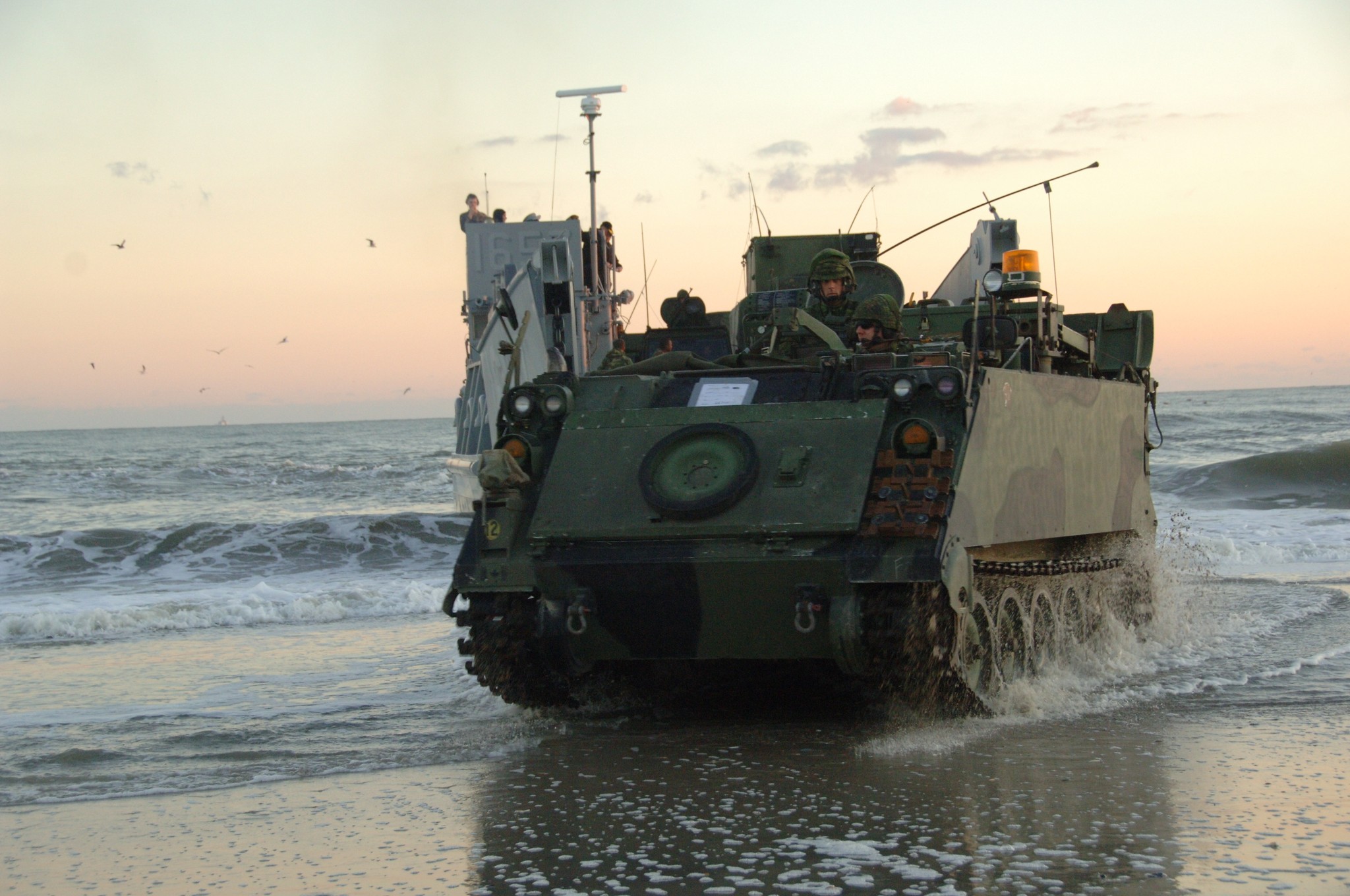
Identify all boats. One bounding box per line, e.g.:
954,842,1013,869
445,83,639,514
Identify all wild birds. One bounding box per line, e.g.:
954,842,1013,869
64,224,421,403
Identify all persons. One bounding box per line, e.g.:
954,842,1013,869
493,208,507,223
850,294,927,369
667,289,707,327
582,221,623,299
650,337,673,358
763,248,861,366
460,193,488,234
596,338,633,371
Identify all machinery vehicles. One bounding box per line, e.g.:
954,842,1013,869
436,159,1164,718
